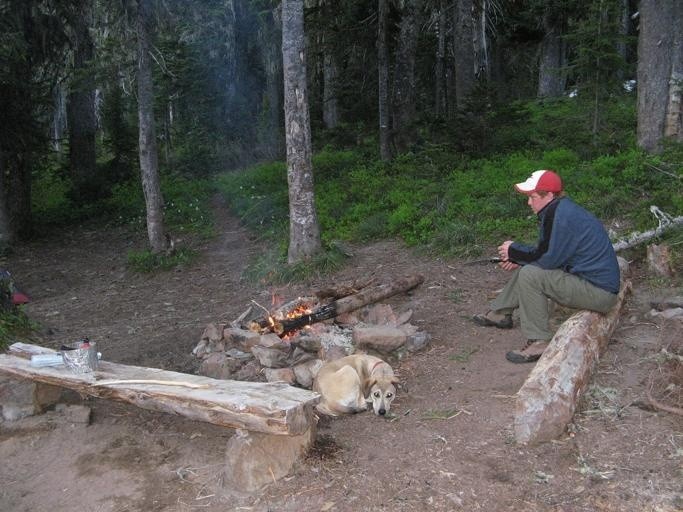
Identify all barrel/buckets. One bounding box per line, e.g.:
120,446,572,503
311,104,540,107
60,342,100,374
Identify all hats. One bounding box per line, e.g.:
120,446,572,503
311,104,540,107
513,169,562,194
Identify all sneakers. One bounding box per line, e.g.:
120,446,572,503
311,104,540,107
507,339,548,363
472,310,513,328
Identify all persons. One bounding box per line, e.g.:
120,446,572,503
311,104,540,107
474,167,620,364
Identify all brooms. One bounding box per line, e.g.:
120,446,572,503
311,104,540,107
11,287,30,305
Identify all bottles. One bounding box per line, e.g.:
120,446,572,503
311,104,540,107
80,336,90,349
60,344,75,350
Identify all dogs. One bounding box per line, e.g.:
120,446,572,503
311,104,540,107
311,354,403,418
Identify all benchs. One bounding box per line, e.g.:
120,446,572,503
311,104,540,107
0,342,322,493
